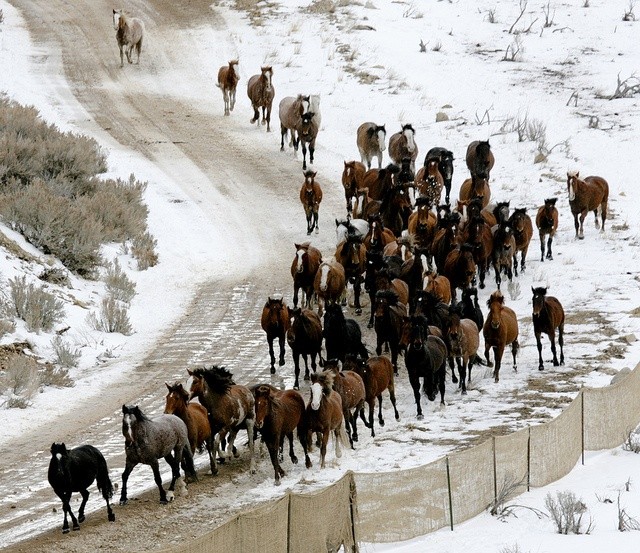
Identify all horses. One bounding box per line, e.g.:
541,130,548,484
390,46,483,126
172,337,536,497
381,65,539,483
447,314,492,397
483,290,521,384
324,300,370,364
382,234,414,263
297,112,319,171
509,206,534,278
413,288,450,337
373,290,409,375
531,285,567,371
335,218,370,250
215,58,241,116
425,147,457,205
493,201,512,225
567,170,611,240
365,159,416,200
466,139,496,174
357,122,387,171
389,123,420,170
459,170,491,208
249,384,313,487
300,170,324,236
287,305,324,392
416,153,445,205
536,197,560,263
341,354,401,439
47,442,116,535
334,233,368,317
119,404,200,506
343,160,367,214
463,196,496,258
279,93,312,152
364,212,397,251
364,251,405,330
291,240,325,310
261,296,293,376
374,268,411,308
398,246,434,302
332,367,367,452
310,93,323,128
444,239,482,290
306,370,353,471
453,285,485,334
409,197,437,245
433,195,465,265
247,65,276,133
184,365,258,476
362,182,414,238
314,255,348,319
112,8,146,68
162,381,227,483
423,269,452,306
493,225,518,291
401,312,454,421
